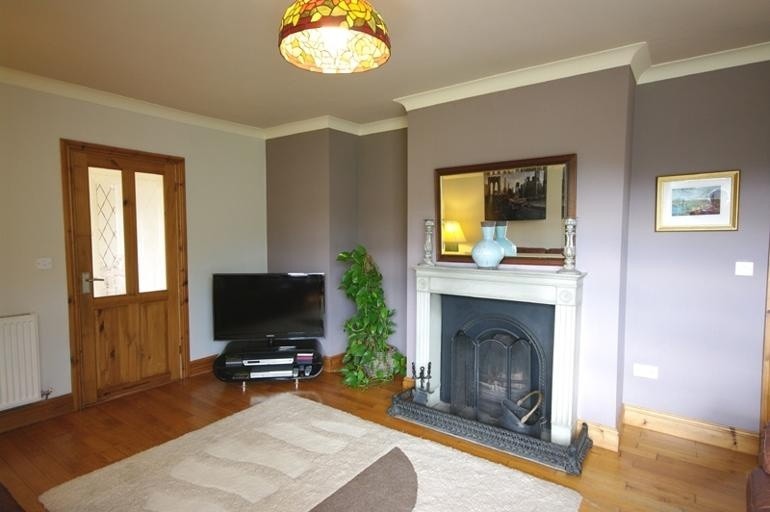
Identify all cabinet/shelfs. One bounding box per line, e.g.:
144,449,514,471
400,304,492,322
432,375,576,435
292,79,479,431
212,351,324,387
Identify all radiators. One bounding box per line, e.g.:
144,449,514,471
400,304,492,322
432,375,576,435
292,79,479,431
1,312,51,414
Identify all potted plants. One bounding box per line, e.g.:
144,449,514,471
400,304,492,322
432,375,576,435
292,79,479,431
335,242,408,391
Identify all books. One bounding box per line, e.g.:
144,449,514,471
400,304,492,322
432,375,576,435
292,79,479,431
296,356,313,360
297,360,313,364
296,353,314,356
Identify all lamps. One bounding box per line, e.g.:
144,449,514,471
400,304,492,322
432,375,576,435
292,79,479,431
440,217,468,253
276,0,393,76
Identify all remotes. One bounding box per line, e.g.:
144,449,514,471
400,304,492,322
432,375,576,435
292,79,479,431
304,365,312,376
300,365,304,372
293,368,298,377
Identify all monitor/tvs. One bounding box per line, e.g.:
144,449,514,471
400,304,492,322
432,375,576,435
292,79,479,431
213,273,325,351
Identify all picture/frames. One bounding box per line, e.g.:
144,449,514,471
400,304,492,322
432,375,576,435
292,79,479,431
654,168,742,233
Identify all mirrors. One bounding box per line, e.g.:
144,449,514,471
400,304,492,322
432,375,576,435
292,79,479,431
432,152,579,268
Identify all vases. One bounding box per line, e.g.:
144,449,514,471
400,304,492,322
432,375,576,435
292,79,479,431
470,220,505,270
496,219,518,260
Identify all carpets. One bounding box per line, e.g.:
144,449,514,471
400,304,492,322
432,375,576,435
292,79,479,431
36,390,585,511
